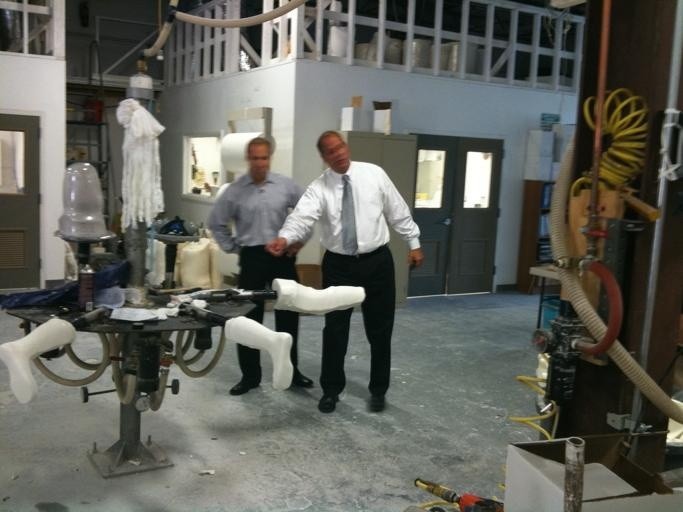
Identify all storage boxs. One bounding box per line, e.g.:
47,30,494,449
500,429,683,510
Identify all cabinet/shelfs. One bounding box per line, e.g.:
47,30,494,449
510,172,554,296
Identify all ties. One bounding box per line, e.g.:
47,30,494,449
342,175,358,255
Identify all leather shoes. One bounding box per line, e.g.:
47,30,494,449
370,395,384,411
319,394,336,412
294,369,313,386
230,377,259,395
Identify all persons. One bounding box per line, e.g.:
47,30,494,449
266,129,425,410
204,138,314,395
